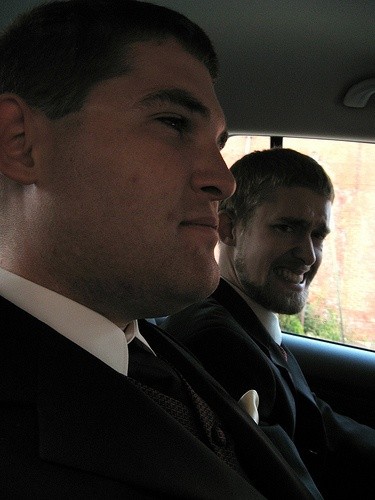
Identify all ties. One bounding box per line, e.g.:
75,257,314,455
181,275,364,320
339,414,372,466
127,340,189,410
278,343,295,373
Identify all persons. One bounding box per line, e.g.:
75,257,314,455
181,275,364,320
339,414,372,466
158,148,375,500
1,0,324,500
